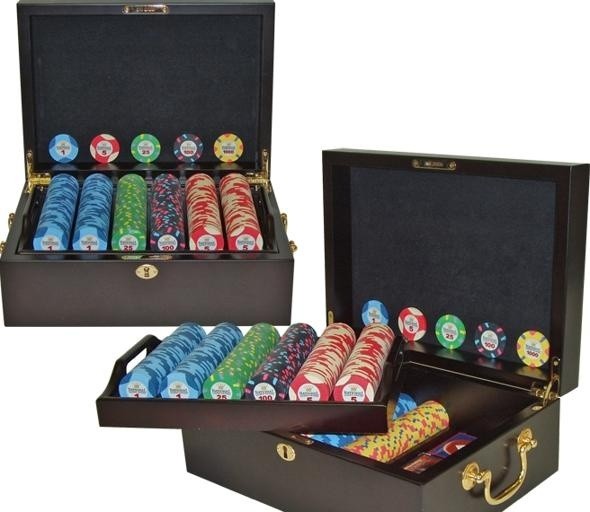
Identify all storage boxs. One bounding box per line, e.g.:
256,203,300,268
95,145,590,512
